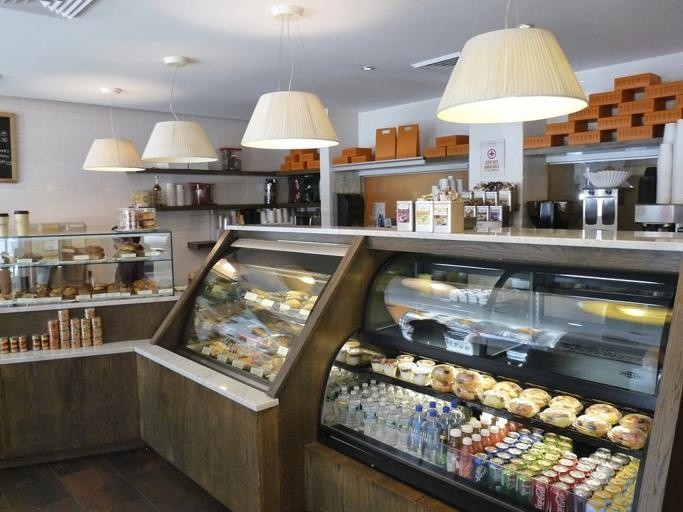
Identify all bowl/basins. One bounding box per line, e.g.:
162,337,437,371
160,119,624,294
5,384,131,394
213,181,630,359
166,181,185,206
216,209,297,228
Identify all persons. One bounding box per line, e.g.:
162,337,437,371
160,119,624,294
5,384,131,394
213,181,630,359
112,226,154,284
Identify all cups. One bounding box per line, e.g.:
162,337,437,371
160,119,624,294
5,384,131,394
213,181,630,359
431,175,464,201
14,212,30,235
654,118,683,206
0,213,9,235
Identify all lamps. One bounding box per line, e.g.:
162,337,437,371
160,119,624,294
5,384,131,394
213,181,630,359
139,54,219,165
240,4,340,151
434,0,589,125
82,87,146,173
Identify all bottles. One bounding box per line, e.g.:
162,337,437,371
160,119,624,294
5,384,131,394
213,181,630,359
263,177,276,204
152,175,162,206
321,353,523,479
377,214,383,227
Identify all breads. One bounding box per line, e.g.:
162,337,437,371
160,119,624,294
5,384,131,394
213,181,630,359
0,278,156,301
136,211,155,229
62,241,146,261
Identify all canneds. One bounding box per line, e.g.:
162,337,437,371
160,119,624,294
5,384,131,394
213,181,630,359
472,427,640,512
265,183,276,205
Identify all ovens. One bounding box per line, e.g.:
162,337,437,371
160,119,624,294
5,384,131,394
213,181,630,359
583,189,632,230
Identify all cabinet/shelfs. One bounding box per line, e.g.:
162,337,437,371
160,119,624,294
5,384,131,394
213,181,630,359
0,227,181,471
131,224,681,512
142,167,322,251
